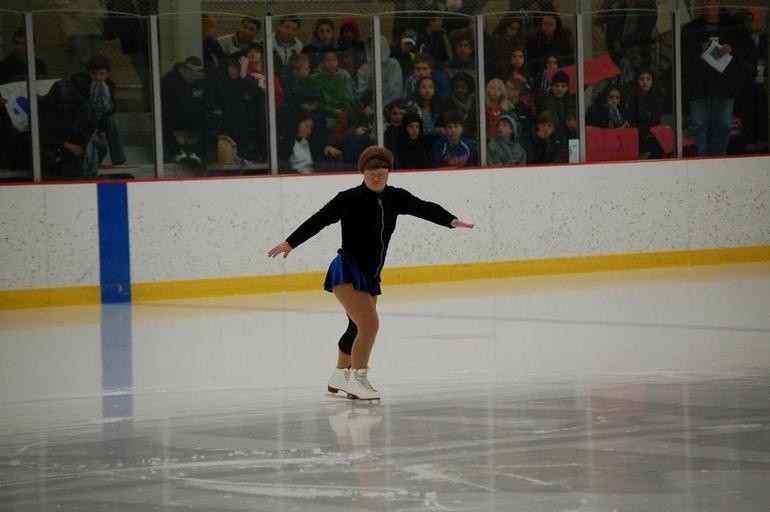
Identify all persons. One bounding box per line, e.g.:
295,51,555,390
328,404,385,511
268,145,473,402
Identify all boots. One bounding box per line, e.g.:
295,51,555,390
327,366,358,396
340,367,382,401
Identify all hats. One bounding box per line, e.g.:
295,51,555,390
550,70,570,84
399,27,418,46
